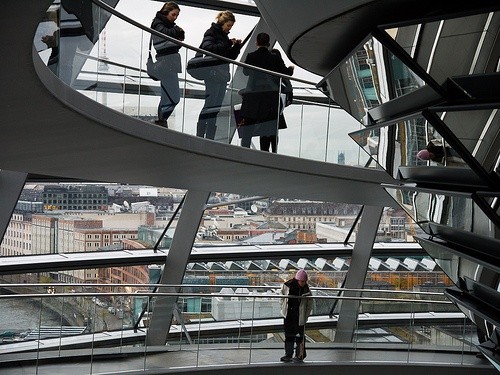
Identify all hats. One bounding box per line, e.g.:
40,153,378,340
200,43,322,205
417,149,430,160
295,269,307,281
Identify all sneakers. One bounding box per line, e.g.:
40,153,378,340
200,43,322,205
290,356,305,363
280,354,293,361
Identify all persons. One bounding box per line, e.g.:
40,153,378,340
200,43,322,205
195,9,243,140
239,31,294,155
41,9,60,74
416,138,464,165
279,269,313,364
151,2,186,128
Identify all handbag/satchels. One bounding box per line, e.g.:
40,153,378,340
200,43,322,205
146,56,174,81
186,55,231,82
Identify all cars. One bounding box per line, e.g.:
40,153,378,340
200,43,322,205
92,297,107,307
108,307,116,314
